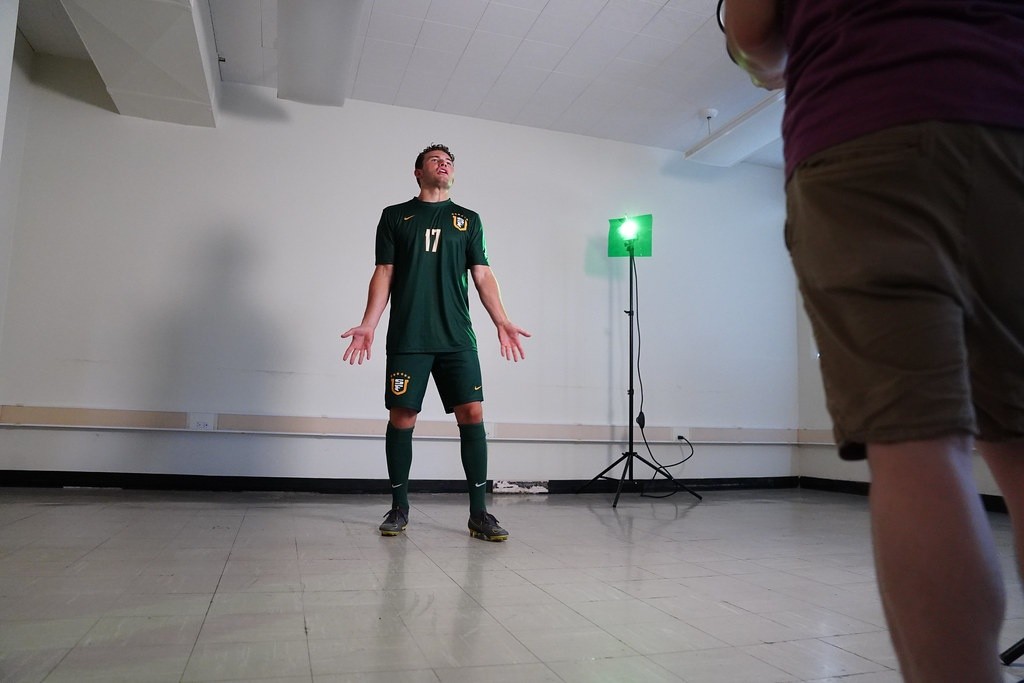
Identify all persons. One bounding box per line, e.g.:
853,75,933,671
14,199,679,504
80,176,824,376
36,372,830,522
337,143,532,544
711,0,1023,683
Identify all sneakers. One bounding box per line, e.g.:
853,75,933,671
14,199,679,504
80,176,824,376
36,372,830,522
378,509,410,535
467,511,509,541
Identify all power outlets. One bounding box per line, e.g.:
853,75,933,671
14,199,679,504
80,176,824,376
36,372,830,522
672,427,690,443
